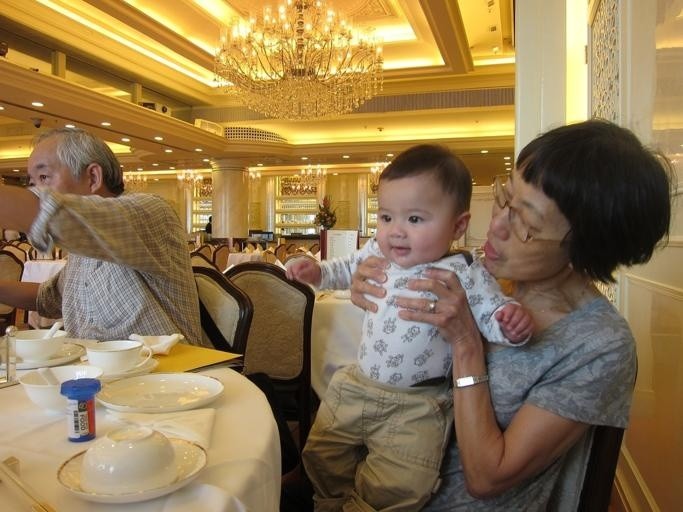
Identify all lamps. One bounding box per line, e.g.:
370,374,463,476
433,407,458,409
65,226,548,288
212,0,385,123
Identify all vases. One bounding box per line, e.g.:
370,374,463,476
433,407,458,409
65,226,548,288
319,224,327,231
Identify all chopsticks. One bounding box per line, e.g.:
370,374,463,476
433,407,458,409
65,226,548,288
0,463,53,512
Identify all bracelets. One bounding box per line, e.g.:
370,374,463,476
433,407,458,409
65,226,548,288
452,375,488,388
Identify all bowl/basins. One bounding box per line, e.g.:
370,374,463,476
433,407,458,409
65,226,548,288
17,365,103,415
79,426,179,495
13,329,68,364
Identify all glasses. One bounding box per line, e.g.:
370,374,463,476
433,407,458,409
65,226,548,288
492,173,572,244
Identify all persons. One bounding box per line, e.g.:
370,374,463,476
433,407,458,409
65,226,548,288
283,142,532,512
1,125,203,347
417,117,672,512
206,215,212,234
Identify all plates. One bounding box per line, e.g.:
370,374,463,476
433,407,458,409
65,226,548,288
96,371,224,414
0,342,85,370
58,438,207,505
77,359,158,382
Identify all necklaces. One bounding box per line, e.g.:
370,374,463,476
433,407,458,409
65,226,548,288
536,276,591,317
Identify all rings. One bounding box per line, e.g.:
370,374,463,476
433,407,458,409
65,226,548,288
429,301,436,313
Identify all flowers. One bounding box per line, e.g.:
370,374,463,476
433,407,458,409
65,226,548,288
313,196,337,228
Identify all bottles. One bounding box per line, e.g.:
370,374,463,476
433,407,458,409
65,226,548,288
56,378,100,441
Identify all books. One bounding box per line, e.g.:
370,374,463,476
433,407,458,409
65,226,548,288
149,343,244,373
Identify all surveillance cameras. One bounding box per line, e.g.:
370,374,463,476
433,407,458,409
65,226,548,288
31,119,42,129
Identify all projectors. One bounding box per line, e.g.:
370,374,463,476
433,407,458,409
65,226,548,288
138,101,172,117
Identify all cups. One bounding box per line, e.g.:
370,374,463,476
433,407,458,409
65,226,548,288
279,200,316,235
86,339,152,372
368,196,380,235
